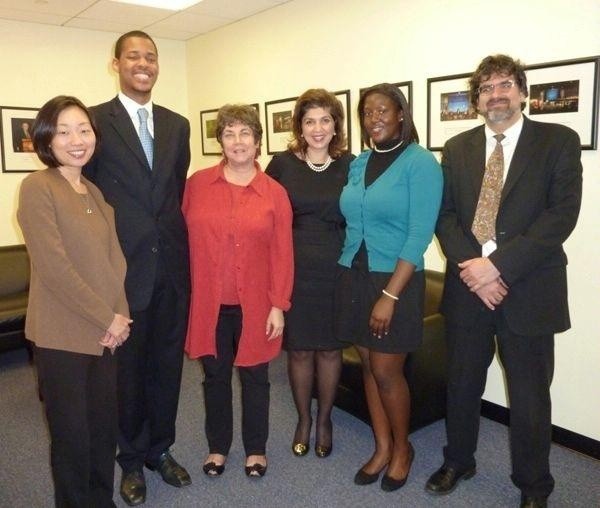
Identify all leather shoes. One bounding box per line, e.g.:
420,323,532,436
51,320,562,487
314,416,334,459
118,455,149,507
424,454,478,495
380,441,416,493
243,452,268,480
292,415,313,457
516,487,550,508
144,449,193,489
354,448,391,487
202,452,228,479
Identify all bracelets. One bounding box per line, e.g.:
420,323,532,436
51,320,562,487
382,288,399,301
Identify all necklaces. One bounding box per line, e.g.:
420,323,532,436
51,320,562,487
79,192,92,214
374,140,403,153
305,156,331,172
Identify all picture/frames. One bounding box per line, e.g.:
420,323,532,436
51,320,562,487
0,103,49,175
264,93,297,157
518,53,600,152
199,106,225,157
426,69,481,151
357,79,413,154
249,102,261,114
329,88,354,154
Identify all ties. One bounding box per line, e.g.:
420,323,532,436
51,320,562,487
135,107,156,173
469,132,507,248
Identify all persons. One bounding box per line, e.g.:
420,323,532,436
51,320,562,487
181,102,295,479
422,51,583,507
16,95,134,508
333,82,444,494
20,122,30,139
82,29,192,507
263,88,354,459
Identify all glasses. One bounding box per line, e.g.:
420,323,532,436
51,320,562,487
475,78,522,96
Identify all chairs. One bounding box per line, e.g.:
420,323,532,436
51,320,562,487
311,265,450,438
0,244,35,366
